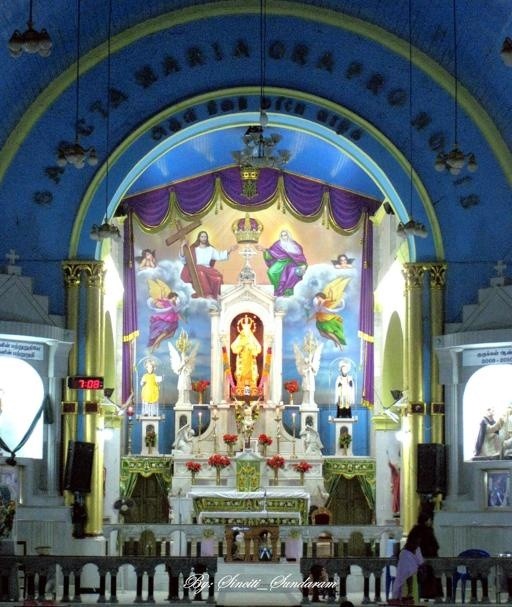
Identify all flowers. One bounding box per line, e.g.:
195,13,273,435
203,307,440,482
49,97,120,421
284,380,299,392
208,454,231,470
191,380,210,393
257,433,272,446
222,433,239,444
292,460,313,473
185,460,201,472
266,455,286,470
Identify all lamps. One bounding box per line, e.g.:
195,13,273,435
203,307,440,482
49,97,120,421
89,2,122,242
392,2,430,242
498,35,511,71
56,0,98,171
433,0,480,178
232,0,293,169
8,1,55,62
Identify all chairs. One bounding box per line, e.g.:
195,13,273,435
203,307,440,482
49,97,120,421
448,546,493,604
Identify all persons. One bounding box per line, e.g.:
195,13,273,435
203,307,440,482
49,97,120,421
471,404,504,457
135,221,378,455
495,404,512,459
400,510,445,601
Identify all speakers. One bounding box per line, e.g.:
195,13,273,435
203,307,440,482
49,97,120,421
416,442,446,493
63,439,95,492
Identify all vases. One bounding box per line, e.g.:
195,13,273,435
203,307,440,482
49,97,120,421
228,444,234,456
198,389,203,403
289,390,294,405
191,472,197,486
274,466,279,484
215,465,221,485
299,470,305,486
262,444,268,457
